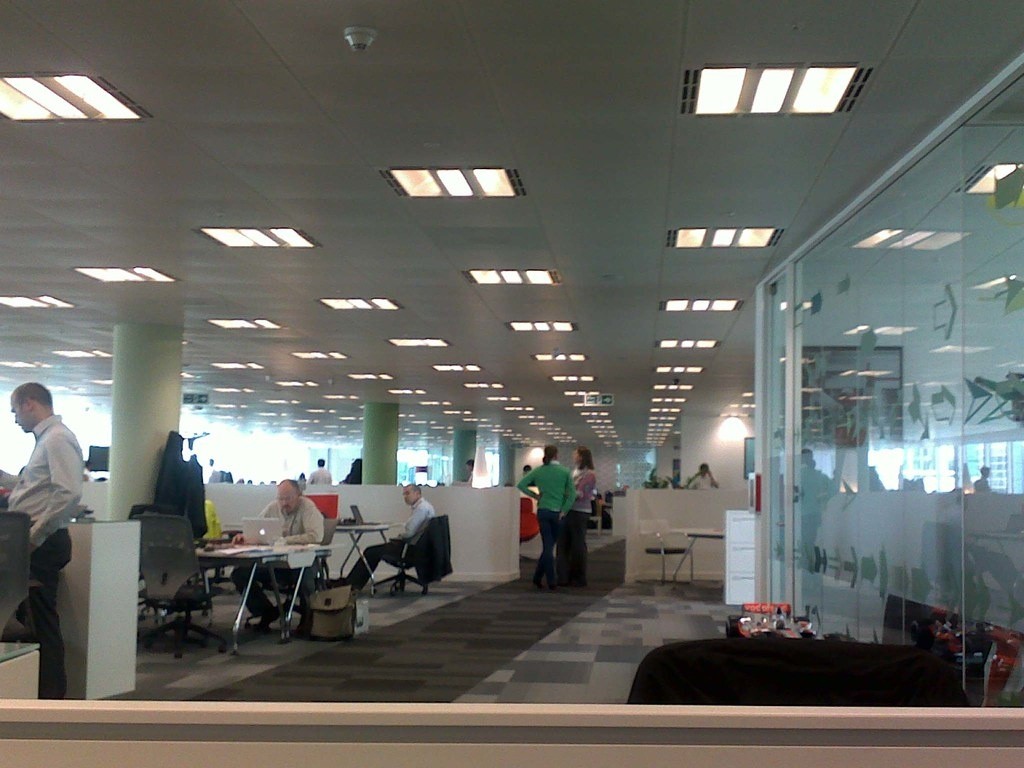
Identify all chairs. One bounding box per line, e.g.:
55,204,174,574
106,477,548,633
370,514,449,595
247,517,338,633
130,514,228,657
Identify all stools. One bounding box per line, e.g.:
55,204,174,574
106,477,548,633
638,546,693,586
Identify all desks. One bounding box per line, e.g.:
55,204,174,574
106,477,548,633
333,523,392,593
673,529,724,604
192,543,334,642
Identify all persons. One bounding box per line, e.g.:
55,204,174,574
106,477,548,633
974,465,990,489
308,459,332,484
518,445,576,593
7,383,83,700
801,449,836,570
232,479,324,640
465,459,474,482
347,484,435,591
688,464,719,490
569,446,597,587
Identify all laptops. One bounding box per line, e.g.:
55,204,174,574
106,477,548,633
240,518,283,545
350,505,381,525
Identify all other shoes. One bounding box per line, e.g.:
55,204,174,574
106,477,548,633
532,582,544,591
329,577,354,591
257,607,279,629
548,584,557,593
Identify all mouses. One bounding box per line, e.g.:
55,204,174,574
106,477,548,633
234,538,243,544
